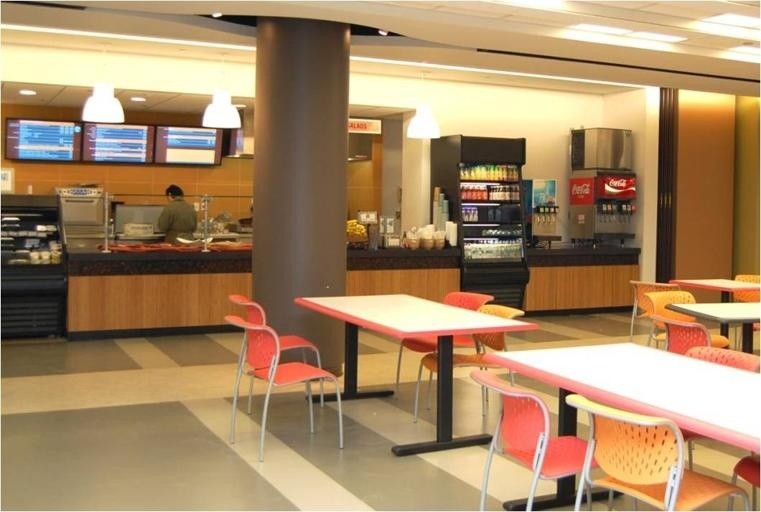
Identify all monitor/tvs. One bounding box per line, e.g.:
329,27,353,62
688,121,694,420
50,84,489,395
154,125,224,167
82,122,155,166
4,117,83,163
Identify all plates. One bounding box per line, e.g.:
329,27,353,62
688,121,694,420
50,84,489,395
177,236,212,245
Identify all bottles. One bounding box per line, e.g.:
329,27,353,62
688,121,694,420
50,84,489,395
462,207,477,221
460,185,487,200
464,240,522,259
489,185,520,201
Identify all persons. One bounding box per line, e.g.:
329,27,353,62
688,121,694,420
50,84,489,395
156,184,196,243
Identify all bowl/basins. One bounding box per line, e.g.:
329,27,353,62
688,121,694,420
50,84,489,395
407,239,420,250
421,239,433,250
435,239,445,249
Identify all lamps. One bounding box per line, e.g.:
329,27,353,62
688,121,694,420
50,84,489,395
202,86,241,130
81,80,127,126
406,103,442,138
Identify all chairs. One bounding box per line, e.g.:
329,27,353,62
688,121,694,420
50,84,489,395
413,304,526,433
733,274,761,331
228,293,325,415
651,313,710,354
469,370,599,511
394,292,495,401
681,430,701,473
729,455,760,511
223,315,344,462
686,346,760,373
629,280,681,341
644,290,729,350
565,394,751,510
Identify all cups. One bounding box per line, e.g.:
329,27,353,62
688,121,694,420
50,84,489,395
30,252,40,260
51,251,64,259
41,251,52,259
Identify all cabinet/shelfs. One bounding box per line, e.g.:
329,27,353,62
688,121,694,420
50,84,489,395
460,177,524,266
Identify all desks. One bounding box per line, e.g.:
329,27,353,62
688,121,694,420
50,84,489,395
294,292,541,458
664,301,761,353
478,342,761,511
669,278,761,350
